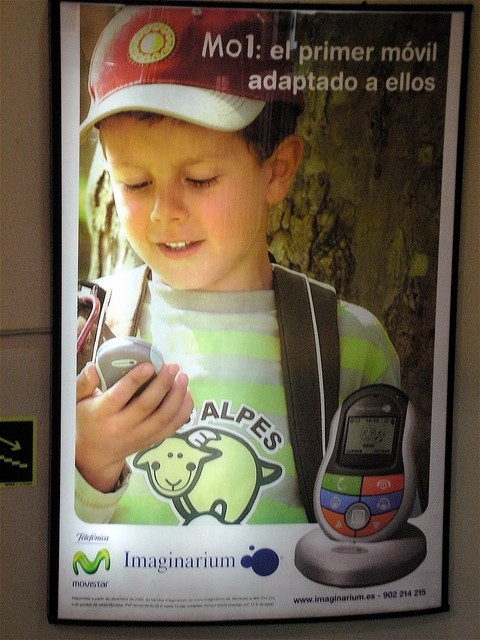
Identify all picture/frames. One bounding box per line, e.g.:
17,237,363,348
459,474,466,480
47,0,473,628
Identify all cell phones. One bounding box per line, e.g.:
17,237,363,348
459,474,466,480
295,386,425,585
94,336,165,396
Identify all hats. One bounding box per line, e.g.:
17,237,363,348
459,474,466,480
79,4,306,134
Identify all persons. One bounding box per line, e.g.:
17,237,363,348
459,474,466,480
74,4,422,524
